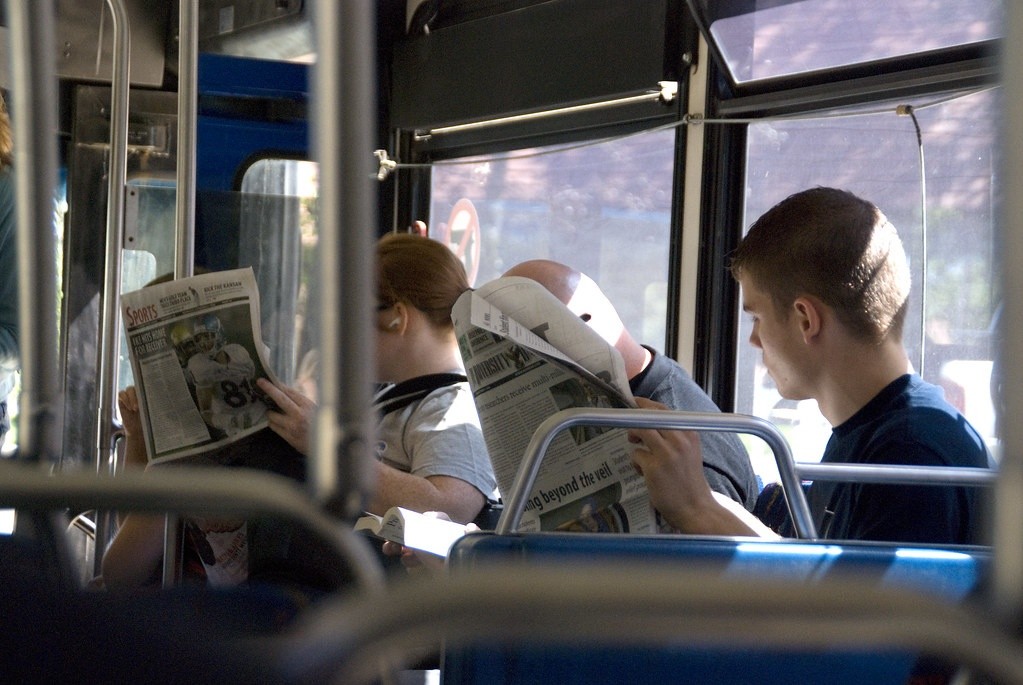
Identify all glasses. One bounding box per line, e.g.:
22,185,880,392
200,333,216,339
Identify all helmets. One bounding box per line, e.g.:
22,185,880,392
194,315,224,334
172,327,195,345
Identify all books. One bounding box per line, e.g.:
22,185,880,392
352,508,481,557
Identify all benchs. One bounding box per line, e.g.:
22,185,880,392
2,455,1023,685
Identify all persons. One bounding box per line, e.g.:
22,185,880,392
581,383,616,432
255,234,497,523
627,187,992,550
382,260,759,567
171,315,273,438
574,501,600,532
100,266,248,598
